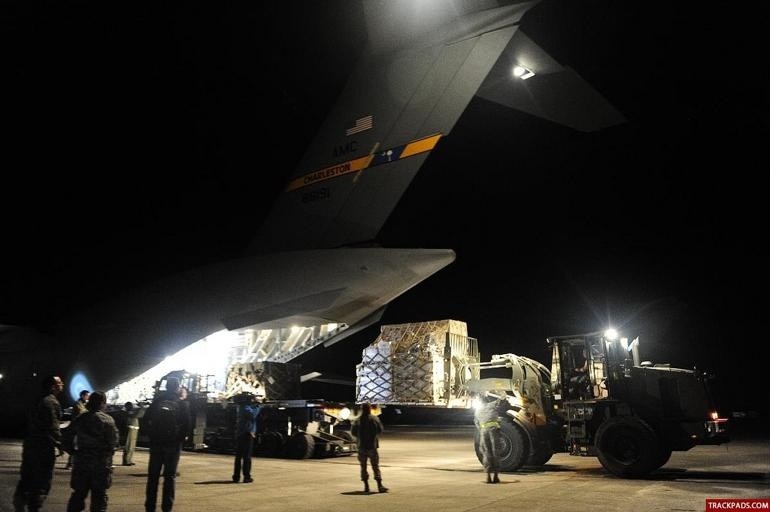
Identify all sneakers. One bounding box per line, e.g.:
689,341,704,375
244,479,253,483
130,463,135,465
122,464,131,466
233,479,240,483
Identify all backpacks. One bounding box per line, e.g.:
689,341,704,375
154,400,179,441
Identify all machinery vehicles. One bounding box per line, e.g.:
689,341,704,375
454,330,728,478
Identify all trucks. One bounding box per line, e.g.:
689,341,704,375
152,367,386,459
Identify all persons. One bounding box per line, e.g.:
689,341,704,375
141,370,187,512
351,403,389,494
473,391,506,484
65,390,91,470
60,391,119,512
232,392,264,483
12,376,67,512
123,402,145,466
162,389,188,477
324,417,337,436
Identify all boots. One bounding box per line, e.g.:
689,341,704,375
486,472,492,483
493,472,500,483
377,479,389,493
364,481,369,493
